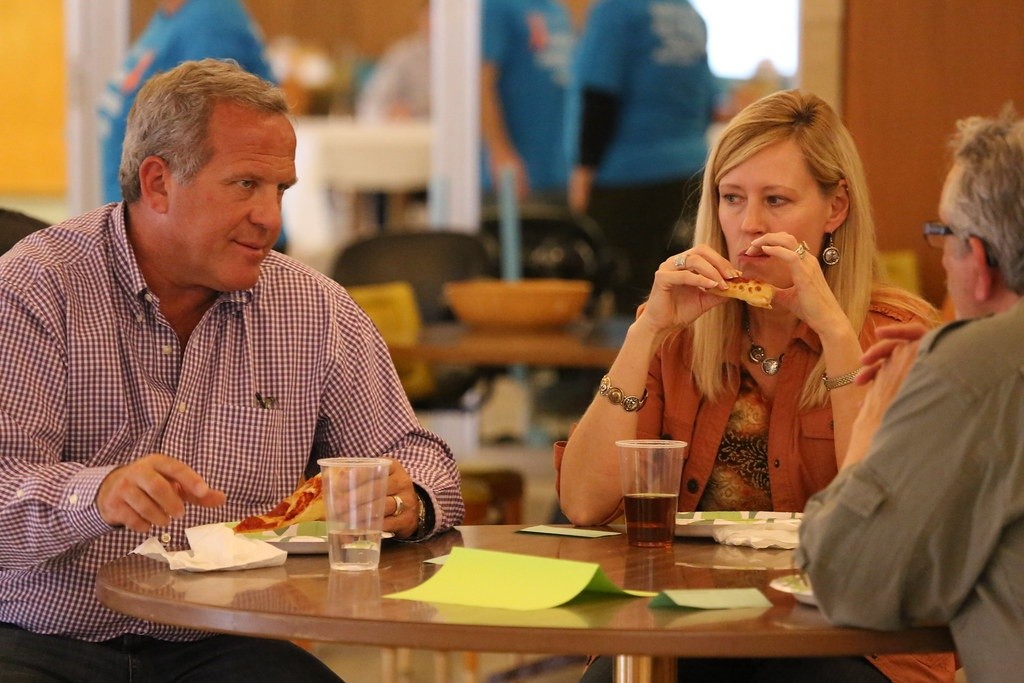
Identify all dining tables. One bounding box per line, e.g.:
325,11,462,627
98,524,959,683
383,324,625,370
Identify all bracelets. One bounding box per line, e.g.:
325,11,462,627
417,494,427,530
822,368,868,388
600,375,648,410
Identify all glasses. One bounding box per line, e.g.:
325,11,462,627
923,222,998,267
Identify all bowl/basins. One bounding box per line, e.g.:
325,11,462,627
443,278,591,327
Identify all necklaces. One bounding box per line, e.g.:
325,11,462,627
744,314,785,375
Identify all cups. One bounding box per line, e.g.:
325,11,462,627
317,457,393,571
615,439,689,548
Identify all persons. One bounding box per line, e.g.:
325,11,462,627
566,0,740,320
554,90,958,682
94,1,289,259
795,109,1024,682
480,0,578,208
0,58,465,683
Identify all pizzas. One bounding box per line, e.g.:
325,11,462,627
233,468,347,534
707,272,775,308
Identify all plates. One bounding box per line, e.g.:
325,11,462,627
674,511,805,538
769,574,817,606
212,522,394,554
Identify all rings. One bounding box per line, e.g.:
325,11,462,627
676,254,688,269
393,495,402,516
796,241,810,259
